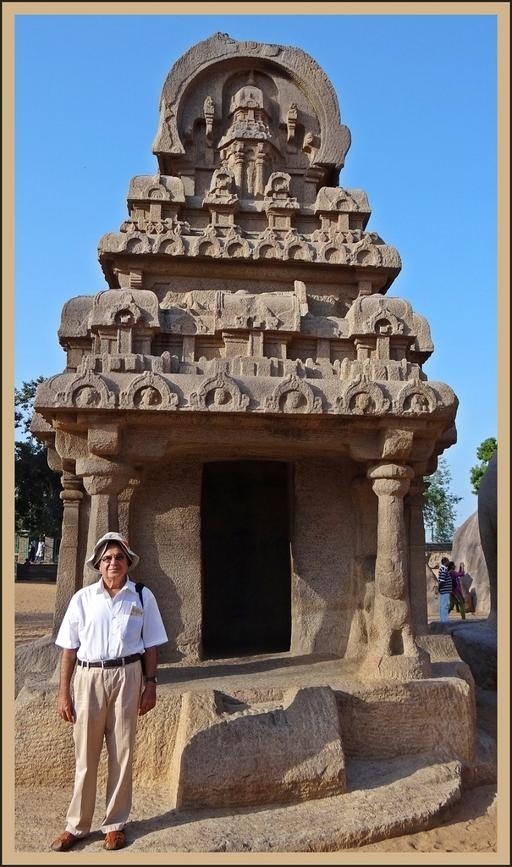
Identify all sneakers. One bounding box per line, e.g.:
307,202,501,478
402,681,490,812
51,831,89,851
103,829,126,850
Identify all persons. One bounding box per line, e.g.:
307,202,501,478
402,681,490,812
49,529,169,853
438,556,452,623
33,538,44,562
447,561,467,621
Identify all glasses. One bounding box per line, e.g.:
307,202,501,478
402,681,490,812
100,553,126,562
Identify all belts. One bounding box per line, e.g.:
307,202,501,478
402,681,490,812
78,653,142,668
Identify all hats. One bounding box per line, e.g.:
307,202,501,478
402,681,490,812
85,532,140,575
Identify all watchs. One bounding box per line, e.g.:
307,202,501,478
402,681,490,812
145,674,160,683
